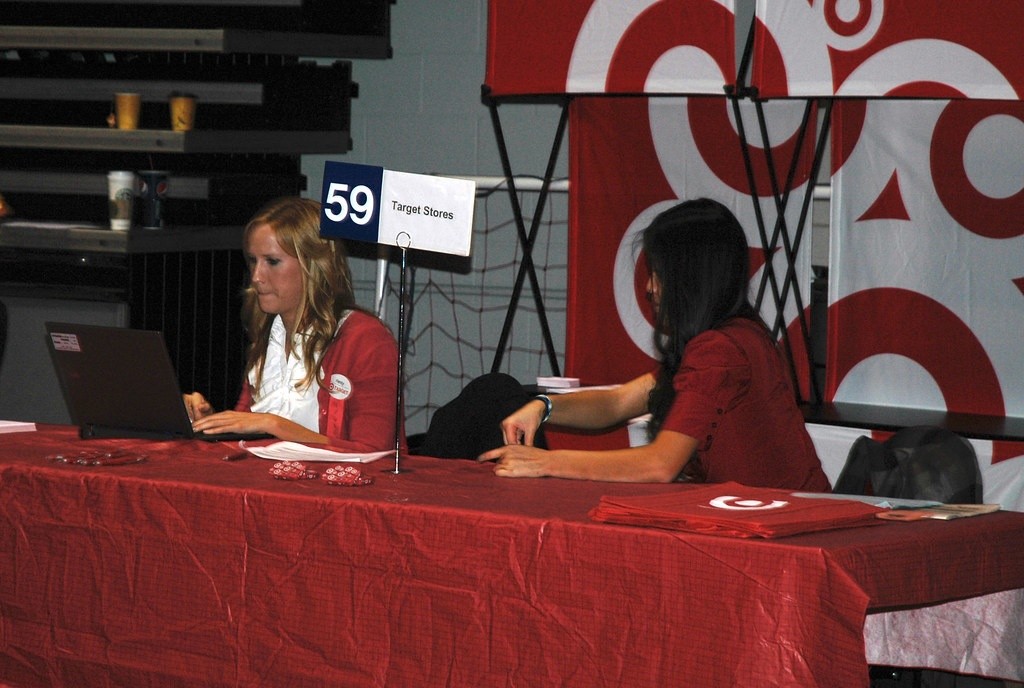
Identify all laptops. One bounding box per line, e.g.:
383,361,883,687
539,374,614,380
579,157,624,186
44,322,277,443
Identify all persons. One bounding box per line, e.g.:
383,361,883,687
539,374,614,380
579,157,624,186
476,198,833,493
177,197,407,458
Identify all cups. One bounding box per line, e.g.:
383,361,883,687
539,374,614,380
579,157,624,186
108,170,135,231
115,92,142,129
138,170,168,230
167,90,198,131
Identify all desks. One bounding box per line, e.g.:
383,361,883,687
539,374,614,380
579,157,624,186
0,423,1024,688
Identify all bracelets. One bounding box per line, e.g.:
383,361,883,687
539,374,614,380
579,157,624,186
534,394,554,423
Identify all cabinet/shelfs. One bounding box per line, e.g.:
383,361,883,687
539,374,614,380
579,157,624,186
1,11,395,260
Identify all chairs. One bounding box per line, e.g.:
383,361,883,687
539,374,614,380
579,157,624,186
402,372,548,469
834,429,984,507
